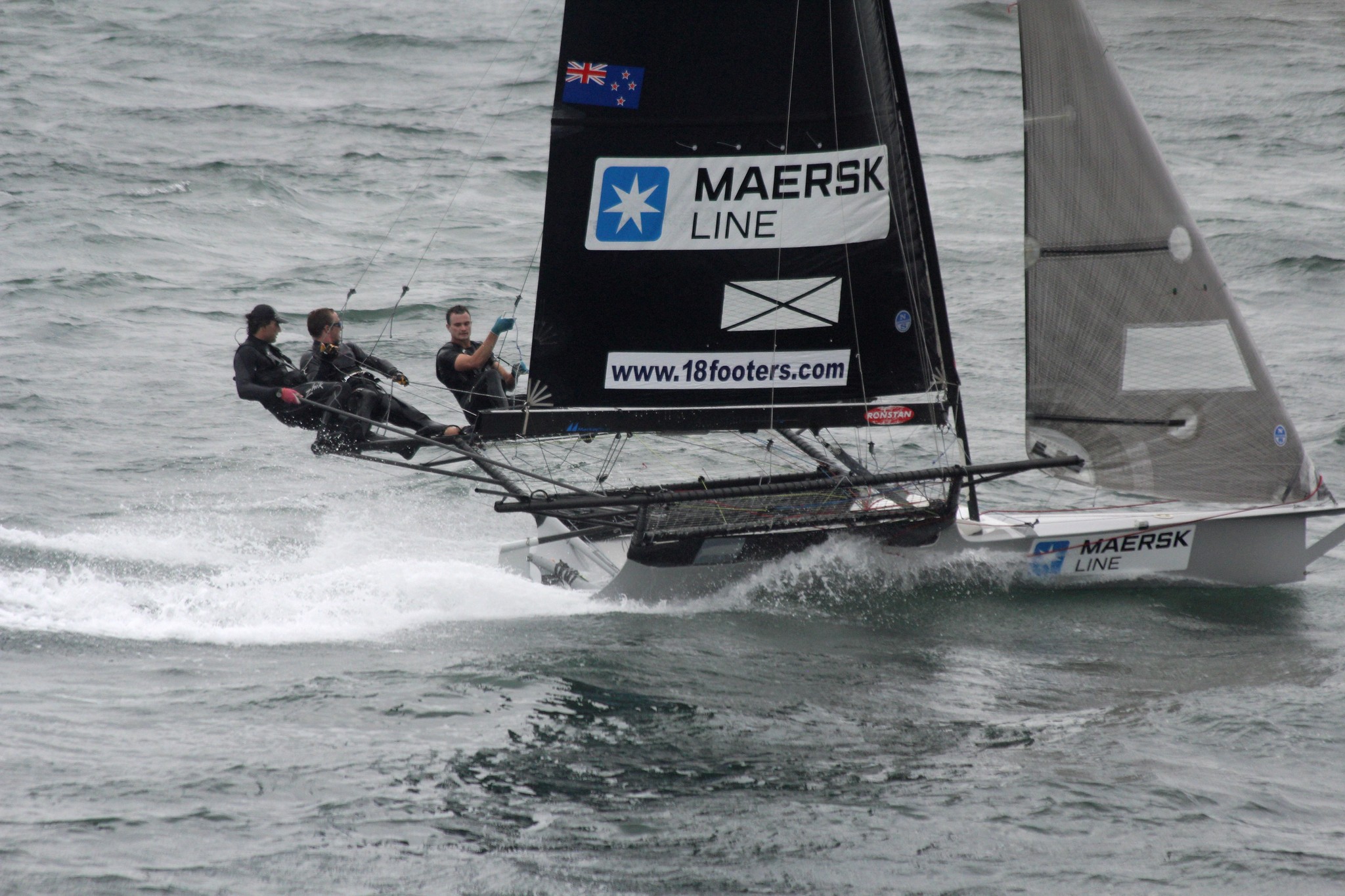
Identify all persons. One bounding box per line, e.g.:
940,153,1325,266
437,306,527,424
233,303,463,455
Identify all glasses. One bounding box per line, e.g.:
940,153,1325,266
323,321,342,330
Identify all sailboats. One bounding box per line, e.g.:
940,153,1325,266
311,0,1345,614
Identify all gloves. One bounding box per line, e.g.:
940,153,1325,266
391,371,409,387
490,311,516,337
318,342,339,365
280,387,303,405
511,361,530,379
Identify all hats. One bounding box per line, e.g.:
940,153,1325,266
249,304,290,323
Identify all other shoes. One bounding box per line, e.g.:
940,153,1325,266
349,423,363,454
578,431,598,444
311,431,350,455
400,426,430,460
